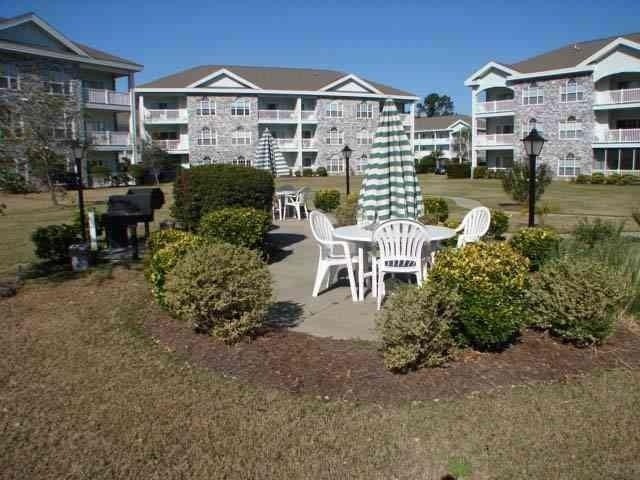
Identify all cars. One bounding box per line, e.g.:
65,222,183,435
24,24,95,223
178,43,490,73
434,164,449,175
133,164,185,185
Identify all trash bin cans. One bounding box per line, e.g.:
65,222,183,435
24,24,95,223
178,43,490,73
465,165,470,177
69,245,89,271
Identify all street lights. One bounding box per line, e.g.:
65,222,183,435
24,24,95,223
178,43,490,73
518,127,547,227
340,145,353,195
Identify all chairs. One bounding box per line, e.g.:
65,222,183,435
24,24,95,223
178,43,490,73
270,185,492,310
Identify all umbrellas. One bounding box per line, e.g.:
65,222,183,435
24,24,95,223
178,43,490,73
253,128,290,178
356,97,426,229
